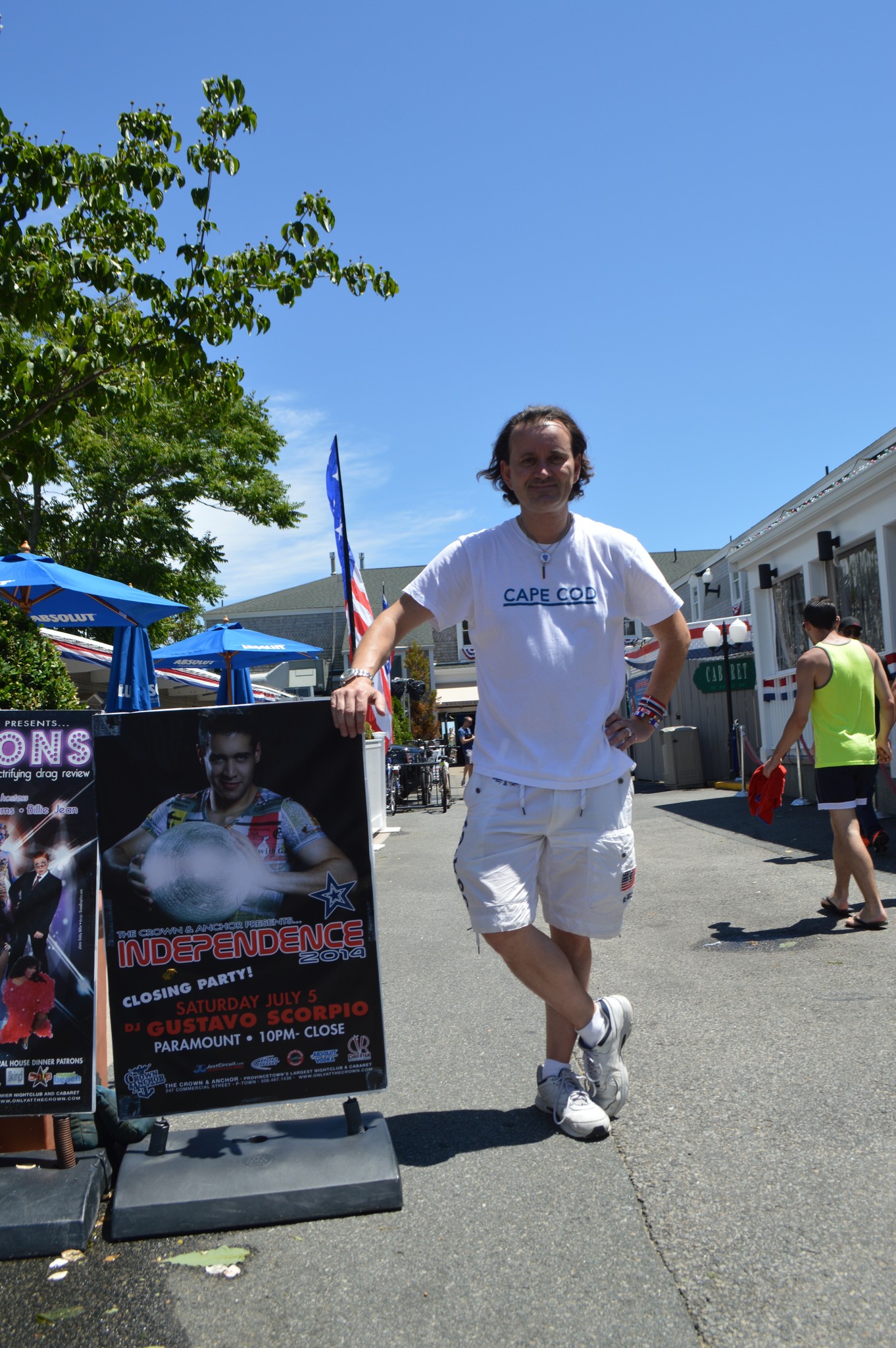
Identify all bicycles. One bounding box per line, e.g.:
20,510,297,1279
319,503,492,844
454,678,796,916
402,738,442,805
386,747,400,816
425,745,461,813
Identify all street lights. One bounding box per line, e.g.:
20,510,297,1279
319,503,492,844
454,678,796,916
703,617,747,781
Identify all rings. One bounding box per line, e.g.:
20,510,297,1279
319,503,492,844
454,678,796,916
625,728,633,737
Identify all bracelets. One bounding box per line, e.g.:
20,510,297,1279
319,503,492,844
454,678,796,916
633,695,666,731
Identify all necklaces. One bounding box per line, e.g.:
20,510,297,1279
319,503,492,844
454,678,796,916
518,511,572,580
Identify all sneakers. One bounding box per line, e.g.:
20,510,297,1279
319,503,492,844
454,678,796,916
535,1065,611,1140
574,994,635,1120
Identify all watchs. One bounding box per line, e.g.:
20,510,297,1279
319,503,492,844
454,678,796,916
338,669,374,687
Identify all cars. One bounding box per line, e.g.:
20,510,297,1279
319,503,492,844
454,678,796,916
387,744,432,788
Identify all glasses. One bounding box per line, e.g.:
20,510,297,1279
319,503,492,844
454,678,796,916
843,630,862,637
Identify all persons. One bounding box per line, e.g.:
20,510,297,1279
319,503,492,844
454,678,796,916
447,716,475,787
761,595,896,930
1,711,357,1065
332,408,691,1141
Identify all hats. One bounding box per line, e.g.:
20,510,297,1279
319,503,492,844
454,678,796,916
839,616,863,632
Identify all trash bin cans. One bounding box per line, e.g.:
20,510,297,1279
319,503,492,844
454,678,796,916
659,726,705,789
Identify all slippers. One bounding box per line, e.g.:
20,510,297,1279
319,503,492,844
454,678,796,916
845,911,889,927
821,897,855,914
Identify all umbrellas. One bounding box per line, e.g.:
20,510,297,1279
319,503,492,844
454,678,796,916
0,540,327,712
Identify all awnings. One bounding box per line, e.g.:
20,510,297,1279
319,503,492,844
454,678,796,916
435,686,479,709
